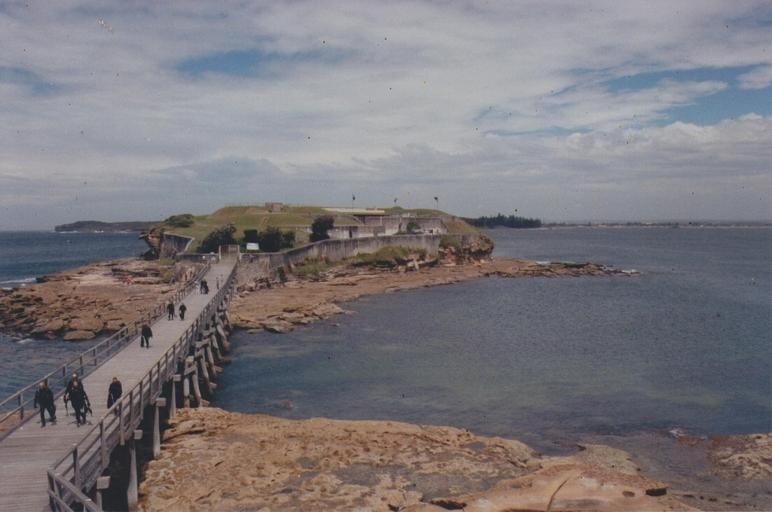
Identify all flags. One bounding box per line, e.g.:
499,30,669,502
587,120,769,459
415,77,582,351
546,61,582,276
393,197,398,203
433,196,439,201
351,193,356,201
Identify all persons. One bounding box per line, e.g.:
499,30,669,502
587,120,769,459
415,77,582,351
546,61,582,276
193,278,210,296
32,380,58,429
64,372,85,397
215,275,220,290
140,322,153,348
220,273,224,283
178,301,187,321
65,380,91,427
166,300,175,321
107,375,122,416
